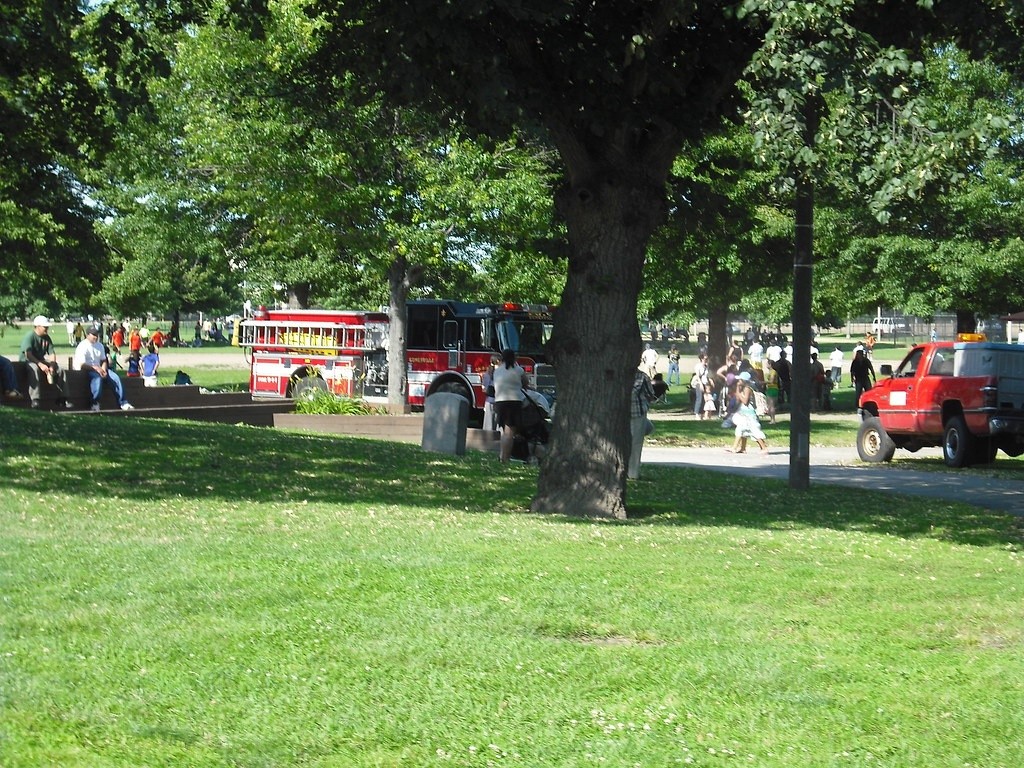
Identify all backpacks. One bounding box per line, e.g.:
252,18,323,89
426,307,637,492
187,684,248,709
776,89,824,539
742,386,769,417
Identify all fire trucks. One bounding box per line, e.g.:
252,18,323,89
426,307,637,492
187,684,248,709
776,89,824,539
236,298,561,416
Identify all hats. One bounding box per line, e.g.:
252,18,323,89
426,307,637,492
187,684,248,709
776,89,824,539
735,372,751,381
34,316,52,326
85,326,99,335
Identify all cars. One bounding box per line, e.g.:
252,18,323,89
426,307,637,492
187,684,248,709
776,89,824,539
726,323,741,332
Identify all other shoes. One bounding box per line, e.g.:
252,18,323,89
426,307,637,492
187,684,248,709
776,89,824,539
119,403,135,410
59,399,74,408
91,401,100,410
4,389,22,398
31,399,41,408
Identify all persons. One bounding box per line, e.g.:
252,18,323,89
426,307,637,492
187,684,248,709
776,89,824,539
911,343,922,370
743,328,787,354
666,343,680,385
931,329,937,342
929,352,945,373
174,371,192,385
853,341,866,386
850,350,876,411
72,326,135,411
195,317,234,345
481,353,502,431
641,343,659,380
66,319,176,387
688,338,844,456
865,332,876,361
0,356,25,401
19,316,75,408
627,368,656,479
1018,329,1024,345
493,349,529,462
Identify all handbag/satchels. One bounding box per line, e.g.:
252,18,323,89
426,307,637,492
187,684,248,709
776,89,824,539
486,385,495,398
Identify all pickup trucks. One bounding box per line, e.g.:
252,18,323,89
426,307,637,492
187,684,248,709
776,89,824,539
855,340,1024,468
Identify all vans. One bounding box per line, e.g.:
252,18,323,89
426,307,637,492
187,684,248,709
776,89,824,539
872,317,911,336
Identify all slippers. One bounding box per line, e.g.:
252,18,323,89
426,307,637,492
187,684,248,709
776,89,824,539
725,449,734,453
736,450,745,453
